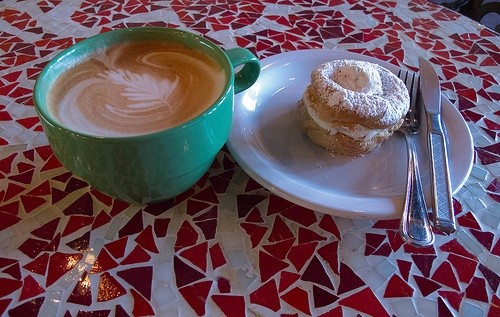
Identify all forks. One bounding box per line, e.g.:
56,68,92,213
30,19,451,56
395,69,435,247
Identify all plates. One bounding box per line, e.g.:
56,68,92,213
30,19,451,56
226,49,475,219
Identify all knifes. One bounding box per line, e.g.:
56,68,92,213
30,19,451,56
417,53,457,233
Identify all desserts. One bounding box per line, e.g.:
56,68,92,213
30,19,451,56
296,59,411,157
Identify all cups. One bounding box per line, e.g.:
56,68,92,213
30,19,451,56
33,26,260,204
